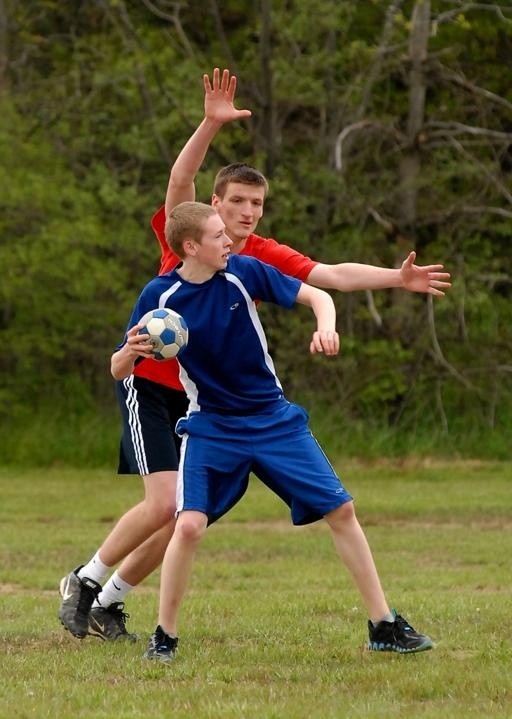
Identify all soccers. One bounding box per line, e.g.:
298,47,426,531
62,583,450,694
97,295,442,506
137,305,191,363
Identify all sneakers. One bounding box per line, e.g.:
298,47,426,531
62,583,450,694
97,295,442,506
88,602,137,643
368,616,433,652
59,565,102,639
142,625,178,664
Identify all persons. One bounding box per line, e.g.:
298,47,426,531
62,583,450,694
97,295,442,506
107,199,438,671
52,65,453,649
